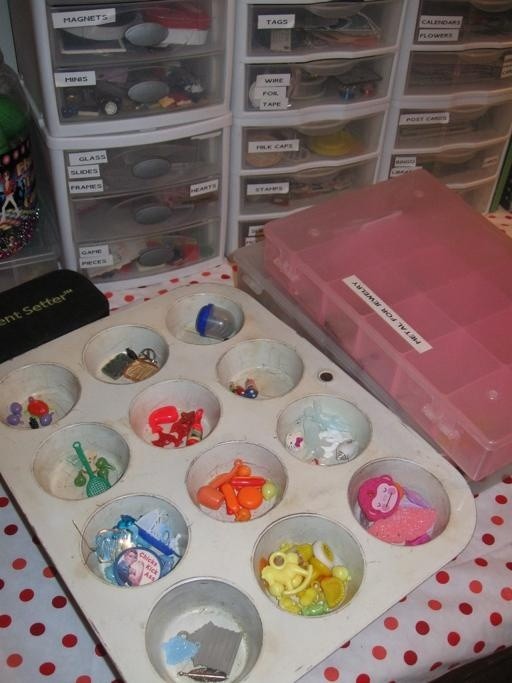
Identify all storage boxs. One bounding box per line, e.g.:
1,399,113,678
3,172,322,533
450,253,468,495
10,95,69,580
1,218,59,291
230,167,512,495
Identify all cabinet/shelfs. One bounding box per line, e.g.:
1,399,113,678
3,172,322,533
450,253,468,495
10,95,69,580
376,1,511,215
230,2,407,256
7,2,229,290
